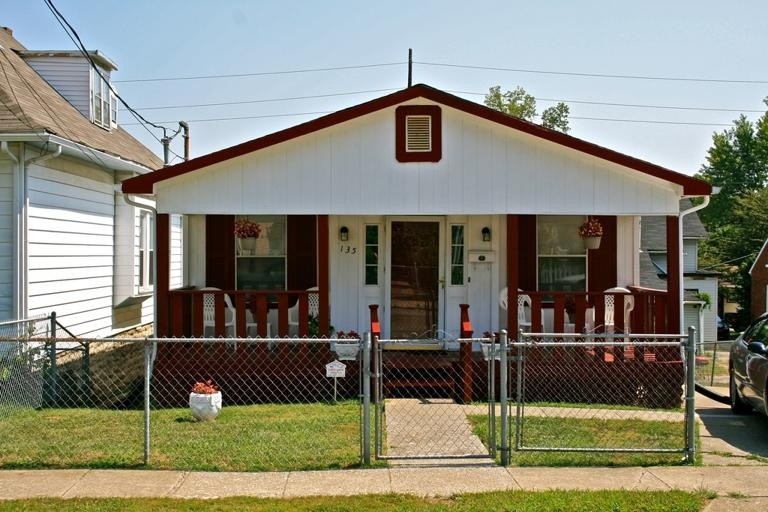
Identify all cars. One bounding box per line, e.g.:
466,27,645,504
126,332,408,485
555,274,587,293
392,281,416,299
717,316,732,339
727,312,768,425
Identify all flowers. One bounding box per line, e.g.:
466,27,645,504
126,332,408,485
577,215,604,240
191,379,219,393
234,219,263,239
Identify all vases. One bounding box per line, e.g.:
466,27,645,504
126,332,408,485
253,313,257,323
240,237,256,250
568,313,576,323
582,237,602,249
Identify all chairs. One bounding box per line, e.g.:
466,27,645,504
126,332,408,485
499,287,546,342
593,287,635,352
200,287,236,354
288,287,319,326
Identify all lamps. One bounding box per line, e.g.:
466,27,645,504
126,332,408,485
341,226,348,241
482,227,490,241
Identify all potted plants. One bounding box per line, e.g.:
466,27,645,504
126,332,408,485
189,391,222,421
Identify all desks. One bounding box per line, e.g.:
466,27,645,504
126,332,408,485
564,324,590,351
246,323,270,352
